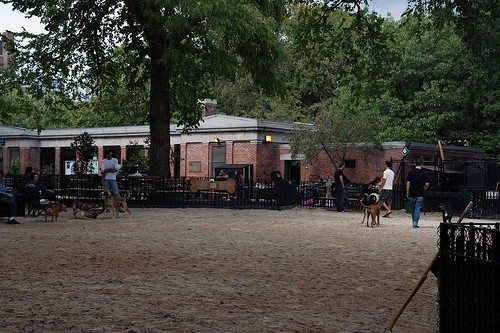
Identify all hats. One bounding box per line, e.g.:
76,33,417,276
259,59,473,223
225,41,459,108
105,147,112,152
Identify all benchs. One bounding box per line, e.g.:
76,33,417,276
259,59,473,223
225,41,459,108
315,187,360,209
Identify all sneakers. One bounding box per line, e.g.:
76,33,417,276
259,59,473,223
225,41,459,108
6,219,20,224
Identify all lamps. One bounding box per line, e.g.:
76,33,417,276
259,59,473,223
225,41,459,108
266,135,271,142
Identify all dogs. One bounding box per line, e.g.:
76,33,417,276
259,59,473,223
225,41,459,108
361,200,381,229
438,204,453,225
44,190,131,223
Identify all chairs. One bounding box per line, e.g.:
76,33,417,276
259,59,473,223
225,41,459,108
26,189,47,217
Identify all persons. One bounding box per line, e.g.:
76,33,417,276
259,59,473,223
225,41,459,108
335,162,347,212
406,158,429,227
101,147,125,214
0,169,20,225
277,170,283,179
26,172,55,203
234,168,244,198
378,161,395,217
128,163,145,191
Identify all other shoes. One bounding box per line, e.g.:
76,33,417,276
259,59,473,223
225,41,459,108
382,210,392,218
413,225,419,228
118,208,125,212
106,209,110,213
